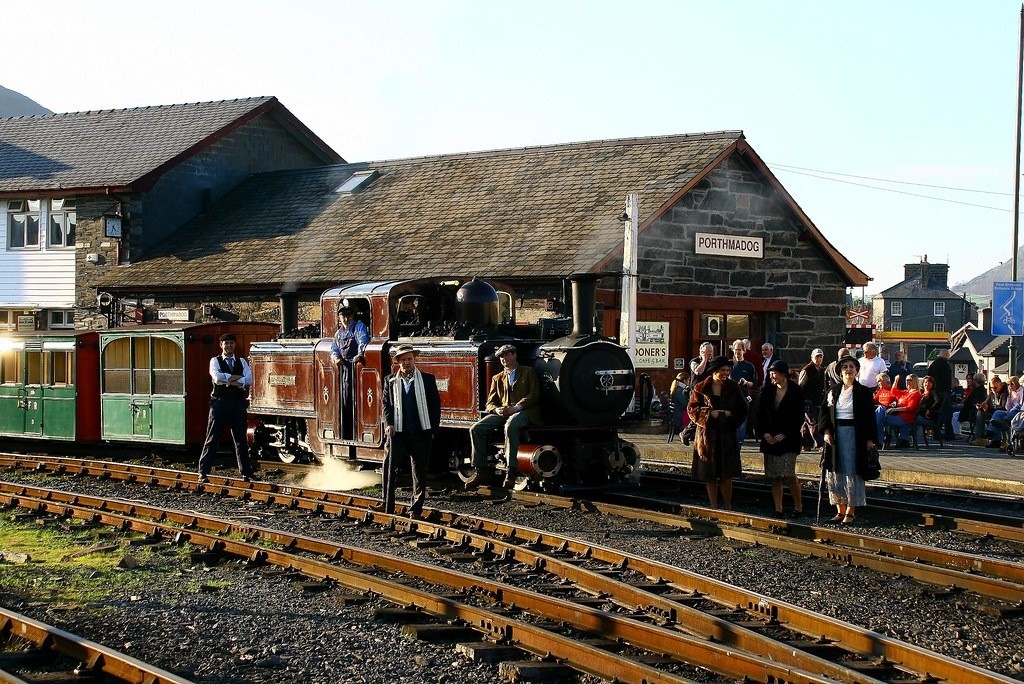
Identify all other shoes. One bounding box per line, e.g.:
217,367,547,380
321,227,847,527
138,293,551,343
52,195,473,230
679,432,689,446
369,504,385,512
969,438,988,445
893,438,910,448
985,440,1001,448
990,418,1009,431
823,513,844,524
955,435,963,440
791,509,803,518
772,510,784,518
983,430,994,440
409,513,419,519
197,475,208,482
841,514,856,526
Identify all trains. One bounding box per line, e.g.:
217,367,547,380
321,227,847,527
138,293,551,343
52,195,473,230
1,271,643,498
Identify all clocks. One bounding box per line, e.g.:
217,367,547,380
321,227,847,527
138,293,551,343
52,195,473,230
102,217,122,239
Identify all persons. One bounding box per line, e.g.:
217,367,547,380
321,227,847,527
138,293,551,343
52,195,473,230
670,337,1024,451
329,307,371,440
368,344,441,519
197,333,259,483
686,356,748,511
465,344,547,490
819,355,882,526
752,359,805,518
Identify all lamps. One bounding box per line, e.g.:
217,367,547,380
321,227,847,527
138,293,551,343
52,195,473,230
456,275,500,302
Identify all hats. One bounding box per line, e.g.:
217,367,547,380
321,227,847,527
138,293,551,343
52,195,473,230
337,307,355,317
219,333,236,340
392,344,420,363
766,359,792,378
835,355,861,378
811,349,824,357
706,356,733,372
495,345,517,357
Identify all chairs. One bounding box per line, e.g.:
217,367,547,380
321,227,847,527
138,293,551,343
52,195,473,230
659,390,994,451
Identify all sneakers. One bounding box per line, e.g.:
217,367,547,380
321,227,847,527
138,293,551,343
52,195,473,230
465,470,491,490
502,470,516,489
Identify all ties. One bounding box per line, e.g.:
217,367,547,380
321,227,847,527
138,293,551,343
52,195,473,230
227,358,234,372
